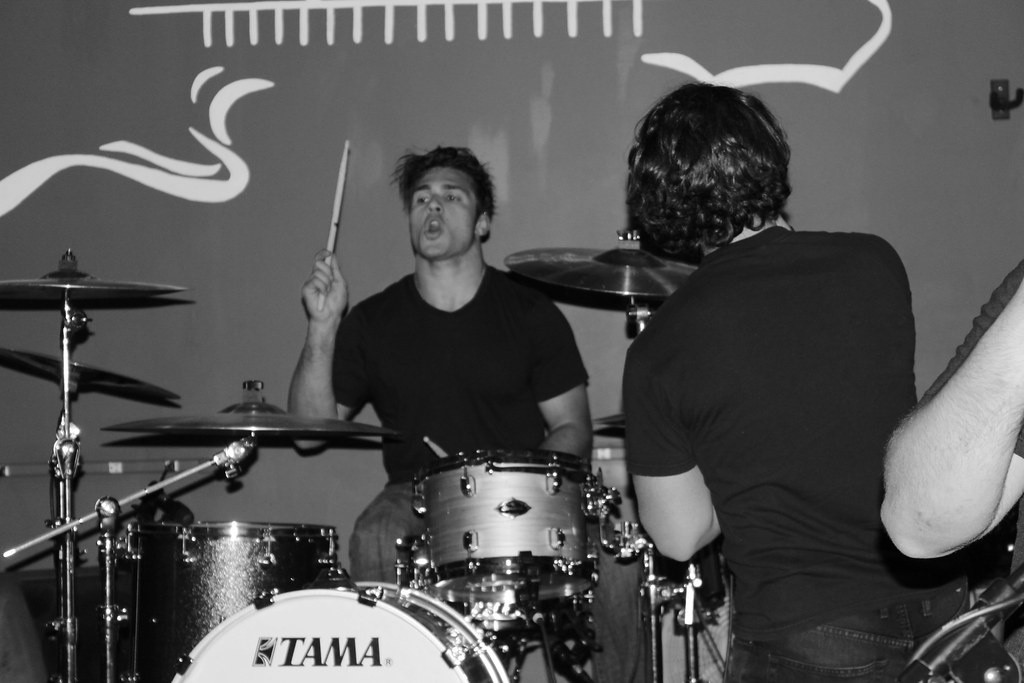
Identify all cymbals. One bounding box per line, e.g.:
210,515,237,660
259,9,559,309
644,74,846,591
0,270,189,300
0,347,182,400
594,413,626,429
503,246,700,297
98,402,405,439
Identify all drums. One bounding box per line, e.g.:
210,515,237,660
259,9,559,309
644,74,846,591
130,518,341,683
167,580,512,683
414,445,601,606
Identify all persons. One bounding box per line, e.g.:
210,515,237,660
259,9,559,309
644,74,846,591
879,257,1024,683
621,82,970,683
287,144,653,683
